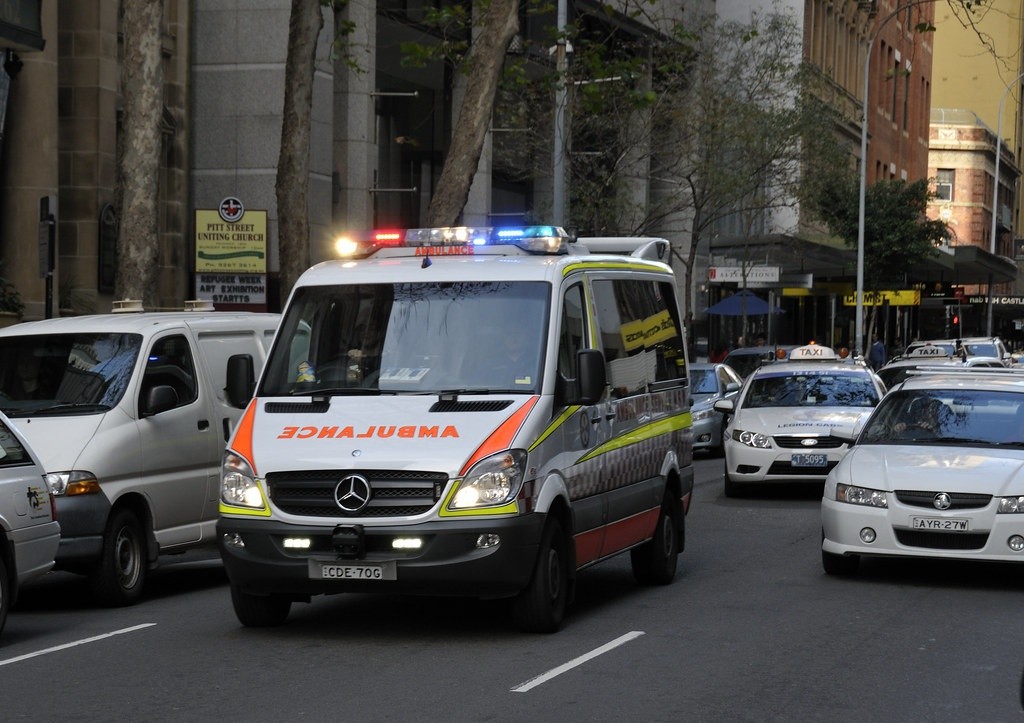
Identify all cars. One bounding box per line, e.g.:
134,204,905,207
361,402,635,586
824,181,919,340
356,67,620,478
723,346,800,382
820,335,1024,586
714,346,885,497
0,409,61,633
688,362,743,454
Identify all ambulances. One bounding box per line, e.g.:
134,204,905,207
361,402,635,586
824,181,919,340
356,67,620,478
215,224,696,635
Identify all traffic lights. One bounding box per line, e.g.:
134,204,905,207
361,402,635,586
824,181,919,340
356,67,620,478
950,315,959,333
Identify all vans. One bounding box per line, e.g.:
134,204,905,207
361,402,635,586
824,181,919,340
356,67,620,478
0,298,311,610
